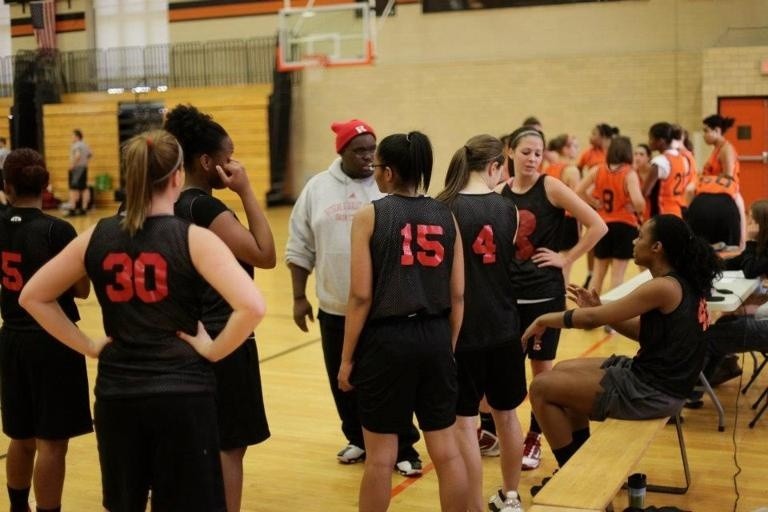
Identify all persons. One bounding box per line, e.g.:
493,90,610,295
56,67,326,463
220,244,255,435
0,137,12,208
60,130,92,212
160,106,276,511
0,148,94,512
18,128,264,512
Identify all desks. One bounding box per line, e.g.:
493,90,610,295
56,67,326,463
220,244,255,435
600,266,760,431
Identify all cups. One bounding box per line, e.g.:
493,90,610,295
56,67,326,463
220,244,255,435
627,472,646,506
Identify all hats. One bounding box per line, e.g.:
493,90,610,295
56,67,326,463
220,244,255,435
332,119,376,153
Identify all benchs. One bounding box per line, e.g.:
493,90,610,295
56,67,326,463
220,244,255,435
519,406,688,512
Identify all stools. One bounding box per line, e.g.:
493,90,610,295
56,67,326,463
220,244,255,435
736,348,767,434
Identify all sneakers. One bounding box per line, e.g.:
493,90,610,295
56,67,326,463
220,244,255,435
337,444,366,464
489,489,524,512
708,355,744,386
522,432,542,470
394,461,422,475
476,429,501,456
